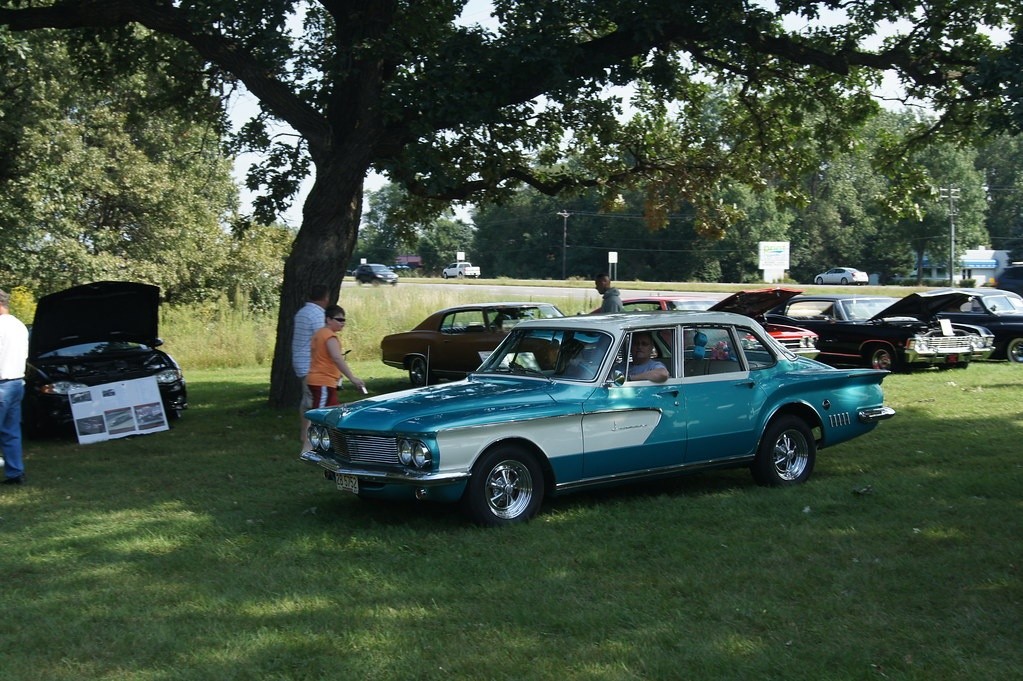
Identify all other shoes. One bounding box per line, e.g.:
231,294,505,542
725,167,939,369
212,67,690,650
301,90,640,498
0,473,27,485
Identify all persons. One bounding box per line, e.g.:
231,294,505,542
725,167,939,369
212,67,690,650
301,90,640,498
0,290,29,485
292,284,331,444
586,274,624,316
300,303,366,461
614,332,671,382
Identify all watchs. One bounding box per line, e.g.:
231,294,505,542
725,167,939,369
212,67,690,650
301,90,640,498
626,374,632,381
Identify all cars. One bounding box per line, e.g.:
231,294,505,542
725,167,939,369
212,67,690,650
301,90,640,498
356,264,398,286
22,280,187,441
619,287,822,360
766,289,997,373
299,311,894,528
814,267,868,285
380,302,564,391
932,287,1023,362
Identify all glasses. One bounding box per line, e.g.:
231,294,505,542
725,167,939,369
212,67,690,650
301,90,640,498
632,341,651,346
332,317,345,323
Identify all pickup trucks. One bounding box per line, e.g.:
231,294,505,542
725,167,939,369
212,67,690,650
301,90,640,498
442,262,480,280
